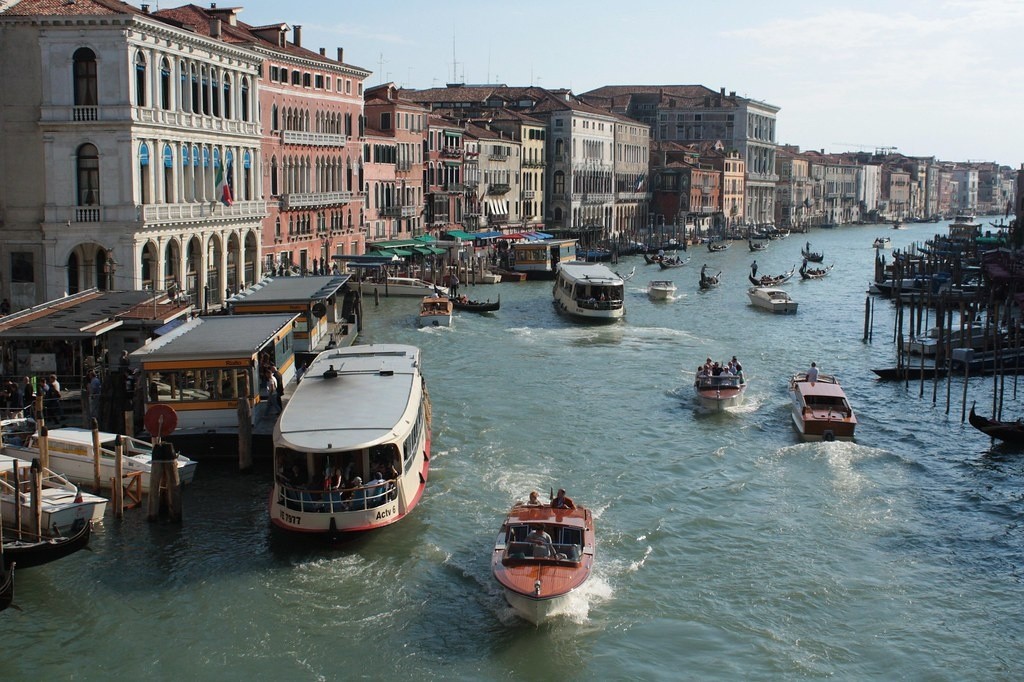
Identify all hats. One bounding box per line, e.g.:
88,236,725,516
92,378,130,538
352,477,362,482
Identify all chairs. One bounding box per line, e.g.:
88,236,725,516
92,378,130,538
507,525,529,544
558,526,582,546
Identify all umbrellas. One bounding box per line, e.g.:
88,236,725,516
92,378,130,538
501,233,523,241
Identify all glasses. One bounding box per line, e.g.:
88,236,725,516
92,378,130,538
534,528,541,531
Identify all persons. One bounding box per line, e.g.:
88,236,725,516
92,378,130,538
526,489,578,509
455,293,480,305
430,290,441,311
701,264,710,281
258,349,284,420
695,355,744,384
812,252,819,258
278,448,400,510
526,523,561,562
807,267,825,275
586,287,619,301
0,343,145,446
802,257,808,272
750,260,758,277
651,248,685,266
448,270,459,298
806,241,812,255
760,274,774,281
324,261,341,276
272,260,310,277
806,362,819,382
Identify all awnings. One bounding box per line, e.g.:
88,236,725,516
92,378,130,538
472,231,504,240
363,246,447,265
446,229,475,241
518,231,553,241
416,233,437,242
369,238,426,249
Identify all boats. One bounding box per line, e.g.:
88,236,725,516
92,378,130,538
268,344,431,546
804,263,835,281
0,452,109,547
707,237,733,252
647,279,677,300
0,416,200,497
748,264,796,287
644,249,677,264
491,499,597,622
552,260,628,323
768,229,791,241
433,284,501,313
747,284,799,315
696,369,747,410
800,247,825,263
418,292,454,329
865,215,1024,381
657,250,692,268
969,398,1024,446
790,370,861,443
749,239,771,252
697,271,722,289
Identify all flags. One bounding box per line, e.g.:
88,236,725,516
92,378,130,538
215,161,235,208
635,171,646,192
